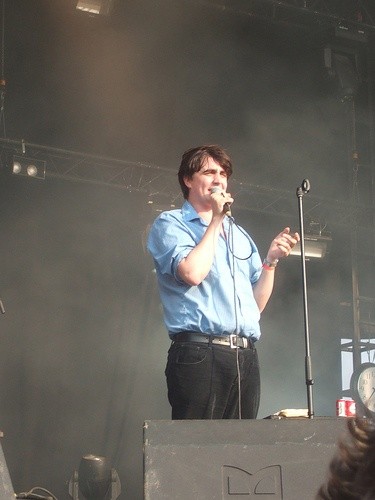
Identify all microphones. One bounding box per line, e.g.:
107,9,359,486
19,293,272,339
211,186,231,218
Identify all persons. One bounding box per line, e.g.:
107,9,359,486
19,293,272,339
146,146,300,419
316,417,375,500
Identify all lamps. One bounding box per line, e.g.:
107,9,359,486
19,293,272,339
9,137,46,181
74,0,106,16
289,208,333,264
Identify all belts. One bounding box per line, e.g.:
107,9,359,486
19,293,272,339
174,330,255,348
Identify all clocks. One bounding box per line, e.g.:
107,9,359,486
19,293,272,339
350,362,375,415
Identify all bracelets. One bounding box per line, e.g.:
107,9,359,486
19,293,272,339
262,257,278,270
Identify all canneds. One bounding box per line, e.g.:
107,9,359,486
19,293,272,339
336,397,357,417
78,454,113,500
